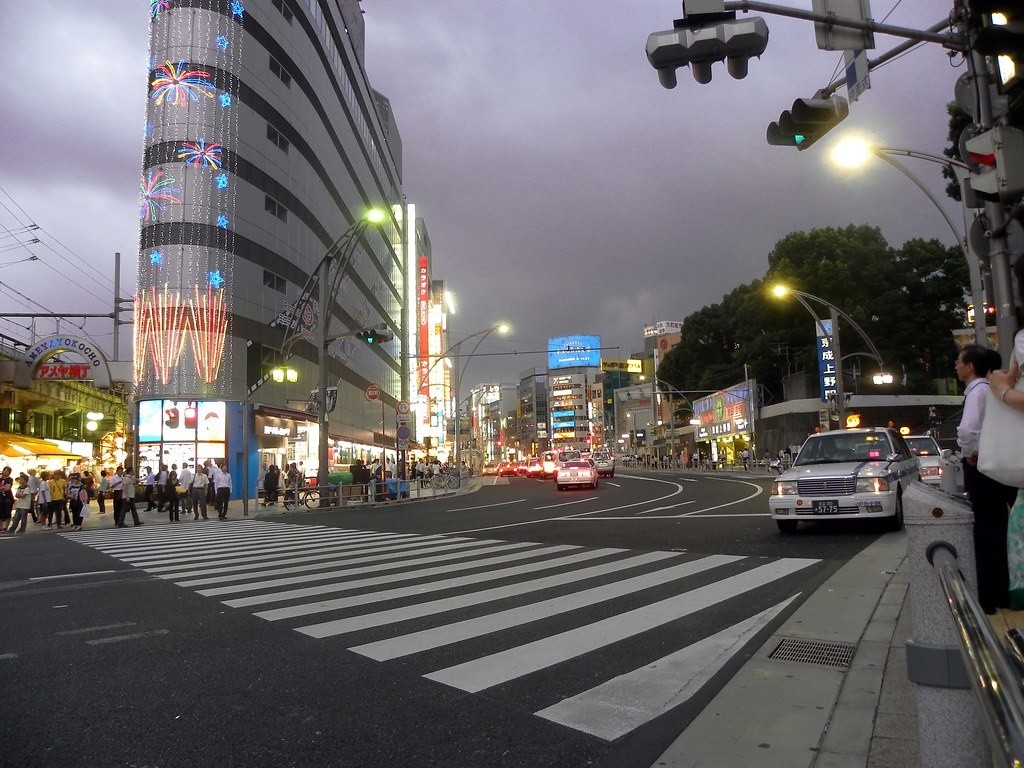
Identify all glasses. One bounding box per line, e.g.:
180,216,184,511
955,360,964,367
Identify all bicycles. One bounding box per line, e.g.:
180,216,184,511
284,480,323,511
423,468,461,489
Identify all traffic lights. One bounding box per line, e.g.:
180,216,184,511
165,408,179,428
184,408,198,429
965,125,1023,201
766,89,849,152
356,329,390,345
644,15,771,90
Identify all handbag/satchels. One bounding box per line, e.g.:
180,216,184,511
185,495,192,509
175,484,186,495
977,375,1024,488
150,490,160,501
79,504,91,518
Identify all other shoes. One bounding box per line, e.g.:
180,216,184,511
40,525,50,530
58,526,63,529
1,528,7,533
72,525,81,532
98,512,104,515
47,523,53,527
6,528,15,534
16,529,25,534
144,508,151,512
158,507,228,522
135,521,144,526
118,524,128,529
153,505,158,509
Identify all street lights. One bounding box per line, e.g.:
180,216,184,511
410,321,510,488
443,385,500,478
830,131,990,351
639,375,702,472
772,281,895,430
270,207,386,508
587,405,630,457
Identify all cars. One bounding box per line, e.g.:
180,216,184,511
902,435,951,484
481,447,615,481
556,460,597,491
767,426,920,534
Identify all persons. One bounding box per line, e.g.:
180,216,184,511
139,460,232,522
737,449,755,472
352,459,409,494
815,439,842,460
110,467,144,528
955,252,1024,615
98,470,109,514
764,450,770,462
264,461,306,507
927,405,936,421
622,450,725,471
409,458,466,488
778,446,792,461
0,467,93,534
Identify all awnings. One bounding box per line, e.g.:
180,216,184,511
0,431,82,460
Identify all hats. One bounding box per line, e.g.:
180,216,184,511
20,474,29,483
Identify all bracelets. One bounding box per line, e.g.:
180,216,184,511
1001,387,1011,406
